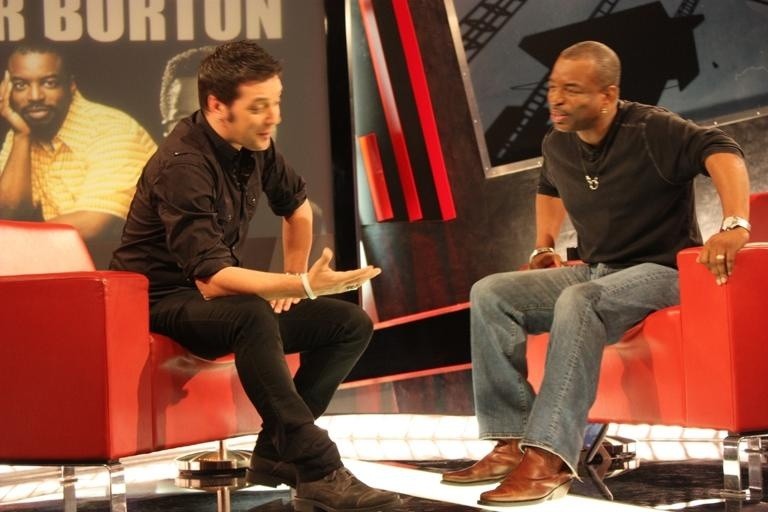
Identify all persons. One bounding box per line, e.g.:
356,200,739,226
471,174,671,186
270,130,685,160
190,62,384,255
443,37,751,506
1,36,159,264
103,41,407,512
161,45,217,140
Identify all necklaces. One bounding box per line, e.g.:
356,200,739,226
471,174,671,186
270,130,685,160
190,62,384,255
571,101,622,193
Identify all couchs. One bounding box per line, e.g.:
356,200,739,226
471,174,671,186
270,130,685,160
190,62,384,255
0,218,301,486
522,190,767,505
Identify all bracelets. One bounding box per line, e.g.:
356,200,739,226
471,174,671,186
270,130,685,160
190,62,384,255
525,245,554,260
284,269,307,277
299,269,320,303
721,213,750,232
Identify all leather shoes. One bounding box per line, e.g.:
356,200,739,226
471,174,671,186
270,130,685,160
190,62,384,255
478,447,570,504
246,451,297,487
290,469,400,512
442,438,526,485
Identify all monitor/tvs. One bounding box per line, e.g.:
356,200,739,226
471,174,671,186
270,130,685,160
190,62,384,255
0,0,362,306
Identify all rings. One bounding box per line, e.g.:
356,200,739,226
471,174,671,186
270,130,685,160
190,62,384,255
716,253,727,262
347,284,359,295
